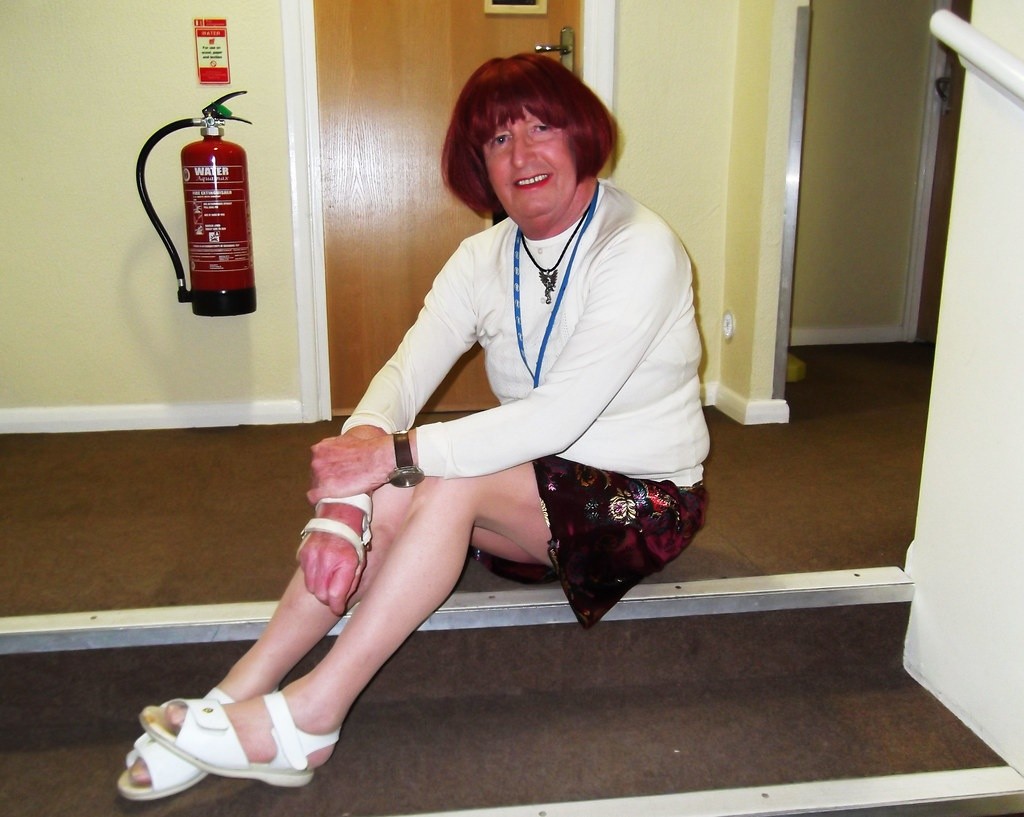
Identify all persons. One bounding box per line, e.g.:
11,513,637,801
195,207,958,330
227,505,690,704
118,53,709,800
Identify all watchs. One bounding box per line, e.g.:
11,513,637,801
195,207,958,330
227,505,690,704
386,430,424,488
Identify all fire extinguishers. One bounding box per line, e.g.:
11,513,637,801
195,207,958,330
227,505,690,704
135,91,258,319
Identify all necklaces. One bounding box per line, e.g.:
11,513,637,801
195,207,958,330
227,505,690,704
513,181,599,388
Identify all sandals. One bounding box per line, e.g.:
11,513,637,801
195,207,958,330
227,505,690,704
139,690,341,787
118,687,278,801
